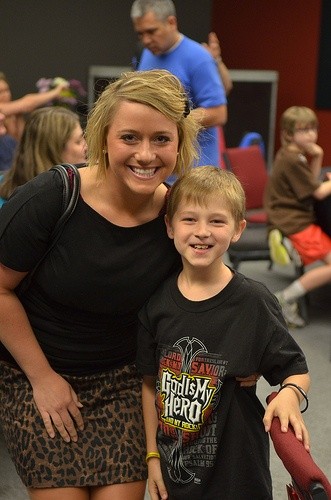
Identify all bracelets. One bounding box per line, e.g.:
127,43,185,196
146,452,160,462
278,383,309,413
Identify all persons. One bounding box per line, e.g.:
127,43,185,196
0,68,202,500
0,72,64,172
264,107,331,329
0,105,88,208
136,164,311,500
202,31,233,92
131,0,228,185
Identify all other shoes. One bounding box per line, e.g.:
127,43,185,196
273,291,304,327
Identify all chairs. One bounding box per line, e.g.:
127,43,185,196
265,391,331,500
226,221,313,325
223,145,271,224
